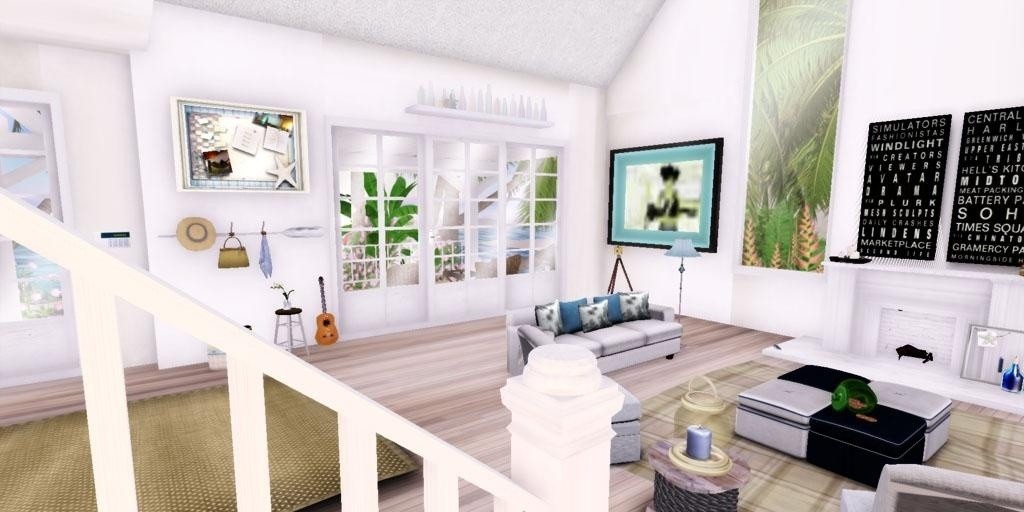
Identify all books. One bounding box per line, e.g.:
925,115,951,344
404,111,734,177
262,125,289,155
231,122,259,156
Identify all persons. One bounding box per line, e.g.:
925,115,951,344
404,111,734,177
654,165,682,230
